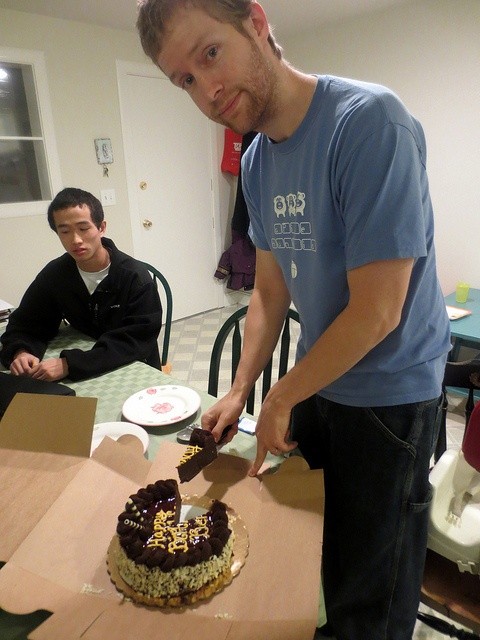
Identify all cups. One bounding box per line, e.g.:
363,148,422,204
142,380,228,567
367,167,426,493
455,281,470,304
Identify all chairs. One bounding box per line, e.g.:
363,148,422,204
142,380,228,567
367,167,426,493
142,260,173,375
209,304,300,418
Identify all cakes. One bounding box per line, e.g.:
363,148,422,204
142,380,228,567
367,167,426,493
175,428,219,482
116,478,232,598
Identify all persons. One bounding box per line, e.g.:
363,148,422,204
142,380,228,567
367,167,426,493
0,189,163,384
135,1,457,639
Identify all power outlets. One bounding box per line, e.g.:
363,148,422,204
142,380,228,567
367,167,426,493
101,190,116,206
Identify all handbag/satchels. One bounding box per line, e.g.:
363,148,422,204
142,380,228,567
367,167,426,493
221,128,243,176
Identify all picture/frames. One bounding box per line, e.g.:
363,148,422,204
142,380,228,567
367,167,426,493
94,138,113,163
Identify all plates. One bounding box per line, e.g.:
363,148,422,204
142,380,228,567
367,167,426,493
122,384,202,428
90,421,149,459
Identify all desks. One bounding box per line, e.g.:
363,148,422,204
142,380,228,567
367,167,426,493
443,285,480,363
